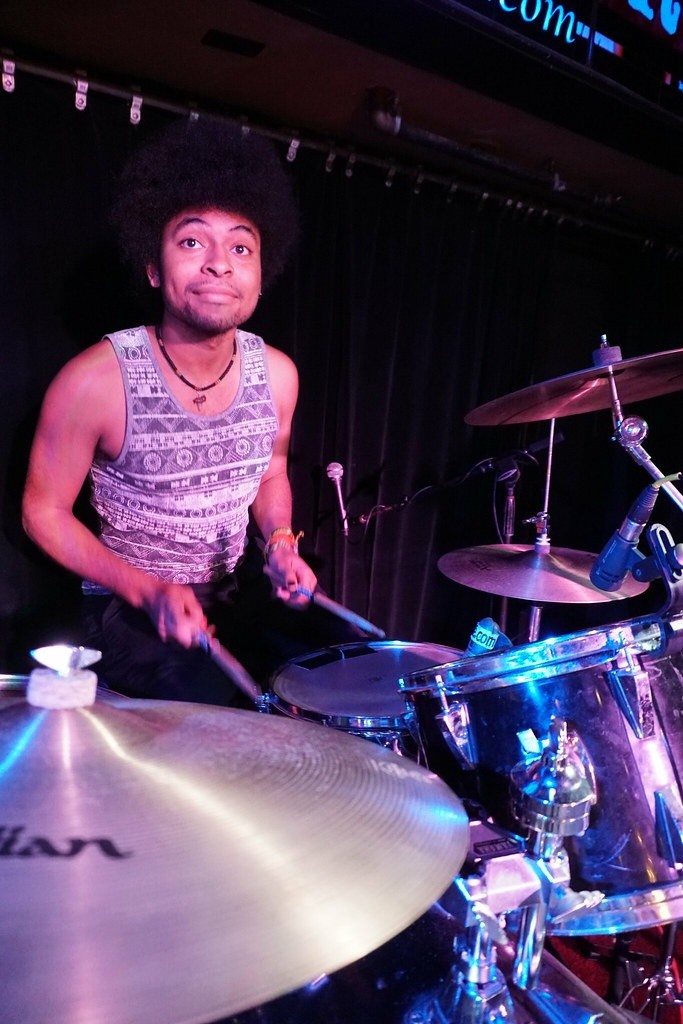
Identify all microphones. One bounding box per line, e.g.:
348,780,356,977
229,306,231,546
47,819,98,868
589,484,661,591
325,462,349,539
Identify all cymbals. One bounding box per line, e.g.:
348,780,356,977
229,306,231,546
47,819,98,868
459,348,683,425
0,644,476,1023
435,543,651,604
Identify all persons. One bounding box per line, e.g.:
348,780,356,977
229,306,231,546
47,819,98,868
21,114,317,706
154,322,237,411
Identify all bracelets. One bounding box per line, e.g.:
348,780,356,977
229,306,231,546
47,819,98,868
264,527,304,565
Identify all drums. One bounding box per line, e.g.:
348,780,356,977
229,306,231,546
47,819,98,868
251,613,683,937
